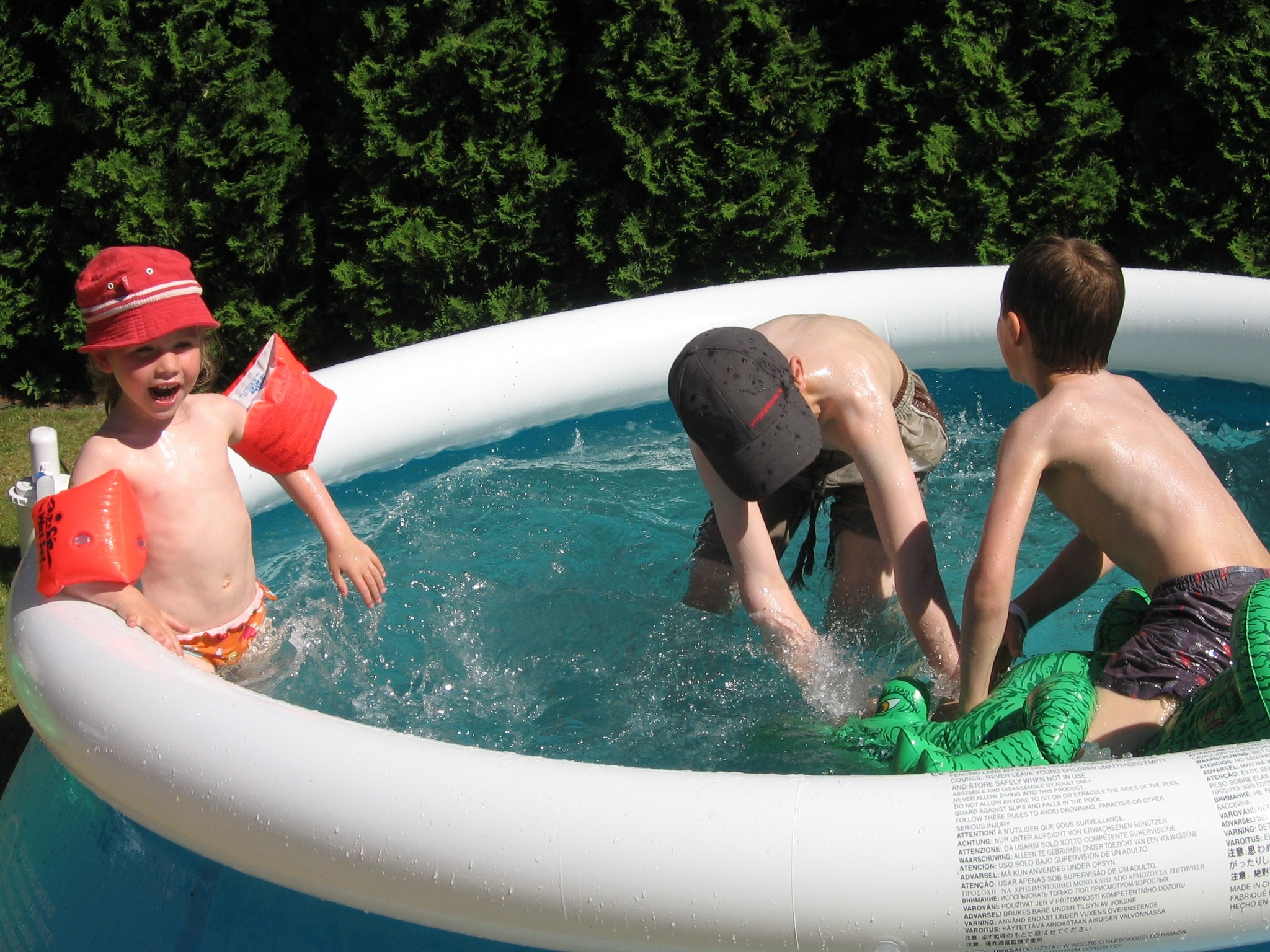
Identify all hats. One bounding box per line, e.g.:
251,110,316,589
668,327,821,502
74,247,221,353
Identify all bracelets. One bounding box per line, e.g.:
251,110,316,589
1008,602,1031,634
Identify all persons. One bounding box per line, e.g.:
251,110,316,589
926,236,1269,756
667,314,969,708
66,247,385,677
